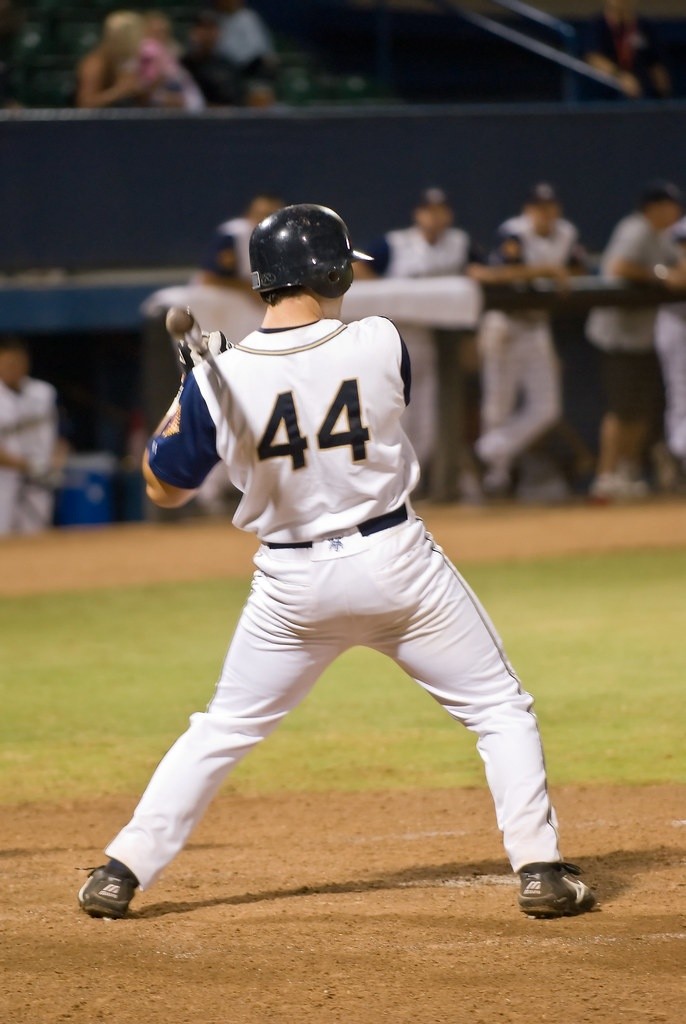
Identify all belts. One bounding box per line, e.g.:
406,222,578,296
267,504,407,548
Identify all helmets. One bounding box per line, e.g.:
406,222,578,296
248,204,373,298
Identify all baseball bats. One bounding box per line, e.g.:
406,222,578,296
165,306,203,347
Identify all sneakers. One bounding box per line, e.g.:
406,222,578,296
518,863,596,915
75,866,135,916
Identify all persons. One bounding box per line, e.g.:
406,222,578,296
585,181,686,499
191,194,282,304
78,0,284,109
0,342,66,536
76,204,595,916
562,0,667,103
475,179,586,503
350,187,486,506
